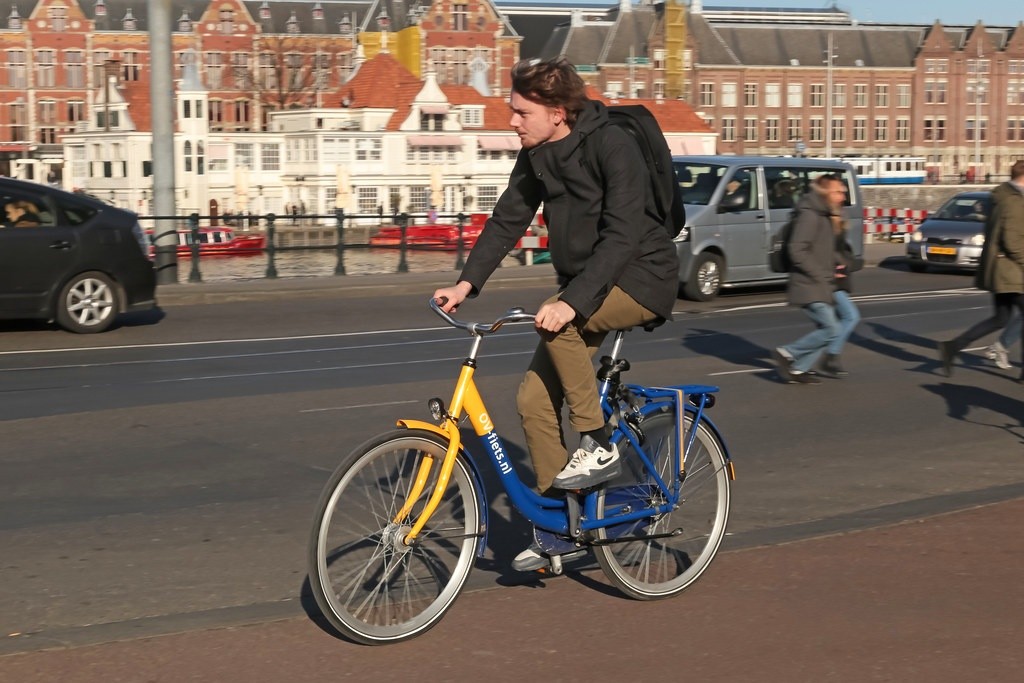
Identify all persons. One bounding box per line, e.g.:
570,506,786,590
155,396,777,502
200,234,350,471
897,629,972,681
0,198,43,228
769,177,803,208
985,314,1022,369
721,173,749,206
937,158,1024,384
770,174,842,384
434,58,680,573
813,178,861,378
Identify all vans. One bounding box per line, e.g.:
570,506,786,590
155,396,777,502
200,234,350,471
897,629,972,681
670,155,867,304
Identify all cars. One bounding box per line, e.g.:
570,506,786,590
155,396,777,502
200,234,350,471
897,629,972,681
0,175,161,335
146,225,265,261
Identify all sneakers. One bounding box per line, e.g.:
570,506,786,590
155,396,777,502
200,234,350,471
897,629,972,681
551,434,622,490
511,536,586,571
982,342,1012,369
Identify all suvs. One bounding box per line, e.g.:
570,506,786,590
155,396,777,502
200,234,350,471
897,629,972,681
906,189,995,275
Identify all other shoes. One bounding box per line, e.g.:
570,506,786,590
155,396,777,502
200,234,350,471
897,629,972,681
788,372,823,385
937,341,954,377
770,348,790,380
817,362,848,374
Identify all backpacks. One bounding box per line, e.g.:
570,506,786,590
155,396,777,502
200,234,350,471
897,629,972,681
768,211,805,273
584,104,686,238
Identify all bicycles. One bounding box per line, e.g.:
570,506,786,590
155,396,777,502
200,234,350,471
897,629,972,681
306,296,736,646
